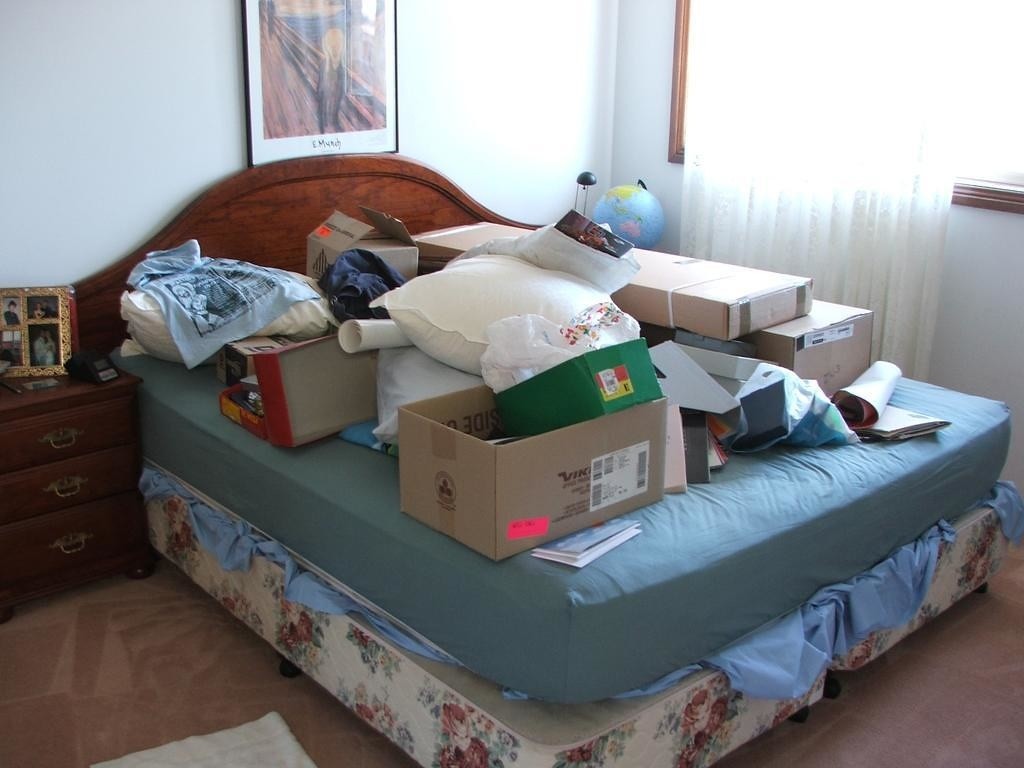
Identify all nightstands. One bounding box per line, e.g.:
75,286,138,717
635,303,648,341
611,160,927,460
0,362,152,623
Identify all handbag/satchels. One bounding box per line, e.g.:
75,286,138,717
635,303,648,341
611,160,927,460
721,364,863,455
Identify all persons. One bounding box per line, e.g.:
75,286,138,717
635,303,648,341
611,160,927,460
32,329,56,365
32,300,52,319
4,300,20,325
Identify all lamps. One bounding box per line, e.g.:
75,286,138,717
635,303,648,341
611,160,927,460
576,172,596,217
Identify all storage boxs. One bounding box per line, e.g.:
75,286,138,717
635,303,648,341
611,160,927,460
217,207,872,563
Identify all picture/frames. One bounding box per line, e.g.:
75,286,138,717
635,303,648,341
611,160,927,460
242,0,399,168
0,286,72,376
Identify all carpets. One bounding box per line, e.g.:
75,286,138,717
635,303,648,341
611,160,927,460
89,711,316,768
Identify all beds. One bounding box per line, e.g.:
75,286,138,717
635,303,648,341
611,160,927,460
70,154,1023,768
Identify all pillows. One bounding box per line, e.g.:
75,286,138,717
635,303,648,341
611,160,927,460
369,252,610,377
120,283,340,365
451,222,644,295
374,346,484,446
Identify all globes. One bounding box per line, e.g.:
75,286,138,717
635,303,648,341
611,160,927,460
591,179,666,250
